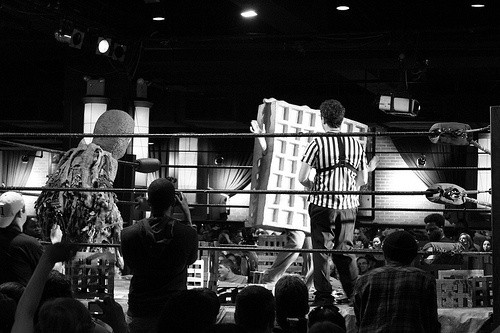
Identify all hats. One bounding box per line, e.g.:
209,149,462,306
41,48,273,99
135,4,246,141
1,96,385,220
382,231,418,263
149,177,177,209
0,190,26,229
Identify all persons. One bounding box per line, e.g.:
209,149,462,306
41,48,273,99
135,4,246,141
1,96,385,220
0,178,493,333
298,100,368,306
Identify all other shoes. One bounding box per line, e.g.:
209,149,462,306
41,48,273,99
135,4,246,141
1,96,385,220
308,296,349,306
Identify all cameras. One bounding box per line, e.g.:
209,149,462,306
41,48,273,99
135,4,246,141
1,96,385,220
88,302,103,315
166,177,182,207
